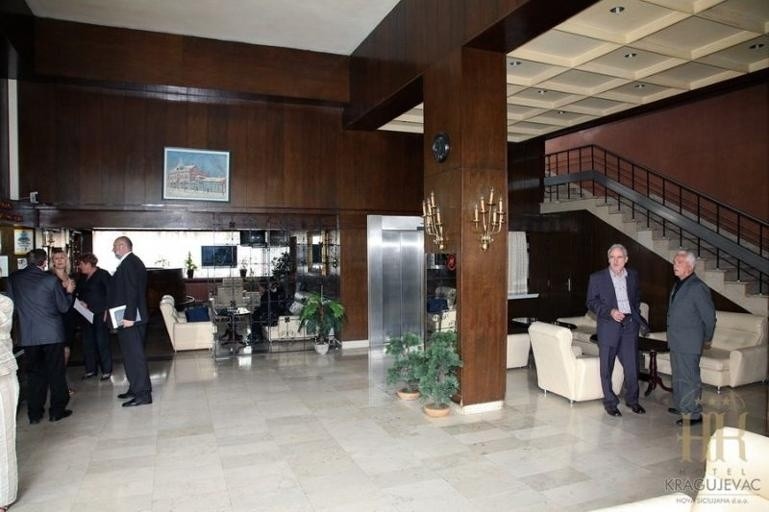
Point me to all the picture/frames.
[13,228,34,255]
[162,146,231,203]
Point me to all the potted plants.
[408,331,463,418]
[184,251,197,278]
[298,292,348,355]
[383,332,423,400]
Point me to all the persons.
[0,291,22,511]
[70,252,113,381]
[248,279,280,344]
[6,248,77,425]
[665,249,718,427]
[106,236,153,407]
[43,251,88,396]
[585,243,647,417]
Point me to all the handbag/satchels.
[184,301,211,323]
[426,295,449,314]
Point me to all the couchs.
[644,311,768,395]
[263,291,335,342]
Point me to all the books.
[107,304,141,329]
[72,297,95,325]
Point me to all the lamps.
[422,189,444,252]
[473,185,506,251]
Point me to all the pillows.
[185,306,210,322]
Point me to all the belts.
[623,313,633,318]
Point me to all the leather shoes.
[122,398,152,407]
[668,407,681,415]
[625,402,646,414]
[82,370,97,379]
[117,390,137,399]
[29,414,45,424]
[247,338,263,344]
[675,415,704,427]
[48,409,73,422]
[100,371,110,381]
[606,407,622,417]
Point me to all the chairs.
[559,302,650,355]
[585,425,769,512]
[160,295,217,352]
[528,321,625,405]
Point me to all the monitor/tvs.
[201,245,237,269]
